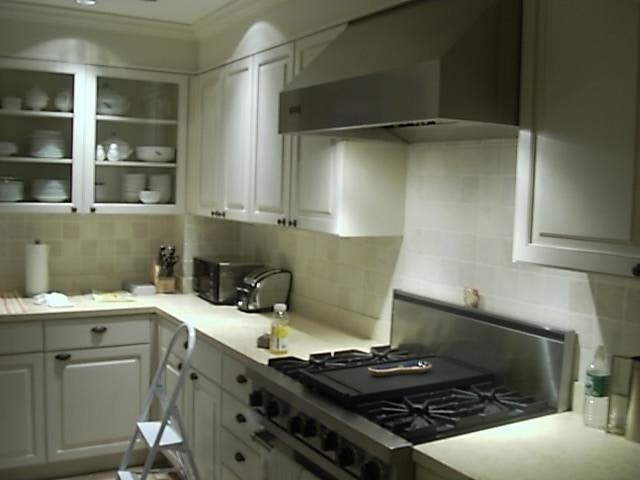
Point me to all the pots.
[96,84,130,117]
[28,128,69,159]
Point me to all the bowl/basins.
[32,177,72,204]
[1,173,27,201]
[136,146,175,162]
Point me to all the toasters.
[234,267,291,311]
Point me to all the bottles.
[106,133,130,159]
[97,144,119,162]
[607,399,624,435]
[578,349,610,429]
[268,303,291,354]
[25,82,72,112]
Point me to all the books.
[91,289,136,301]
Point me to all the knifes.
[158,243,179,270]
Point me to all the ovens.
[250,429,356,480]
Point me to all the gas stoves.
[249,345,548,478]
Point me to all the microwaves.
[192,255,263,305]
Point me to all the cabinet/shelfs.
[0,317,44,479]
[219,349,267,480]
[192,56,252,226]
[43,315,158,478]
[253,25,406,241]
[156,317,219,480]
[510,1,639,282]
[0,54,190,215]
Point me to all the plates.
[122,172,171,203]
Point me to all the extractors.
[277,0,519,147]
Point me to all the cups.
[4,96,20,110]
[624,355,639,443]
[2,141,18,158]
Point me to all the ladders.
[115,322,200,480]
